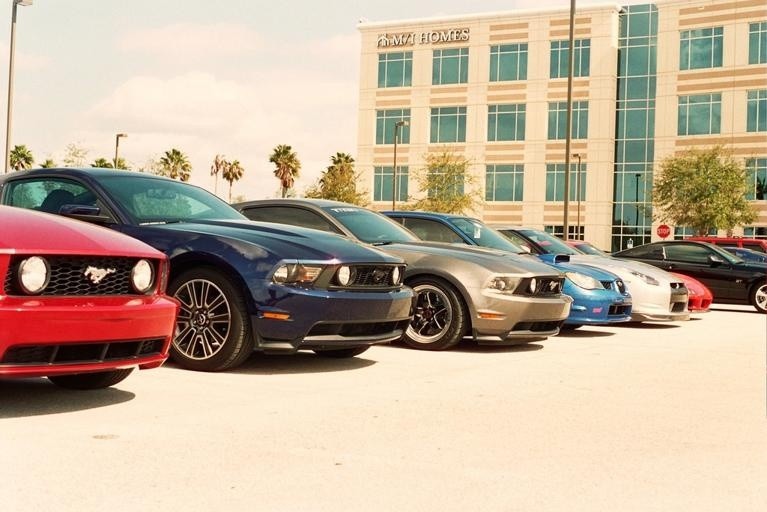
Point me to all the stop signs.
[657,225,670,238]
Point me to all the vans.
[682,236,766,255]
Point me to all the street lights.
[392,121,410,212]
[634,173,641,246]
[3,0,39,173]
[572,152,583,241]
[114,133,129,169]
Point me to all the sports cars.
[0,166,415,377]
[536,242,712,315]
[192,196,573,352]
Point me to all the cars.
[722,246,766,267]
[1,200,181,395]
[496,227,691,323]
[612,240,766,316]
[383,209,635,331]
